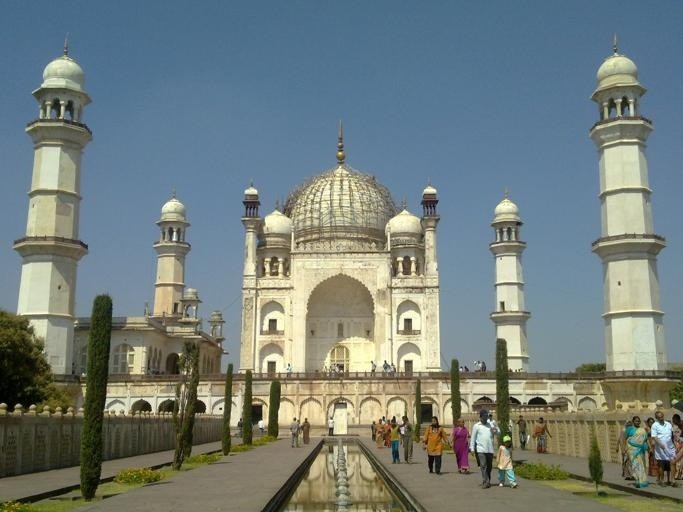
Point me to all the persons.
[289,417,310,447]
[371,360,396,377]
[328,417,334,435]
[258,418,264,435]
[459,360,486,373]
[371,416,415,464]
[286,364,292,377]
[616,411,682,487]
[239,418,243,438]
[508,368,523,372]
[335,364,340,374]
[422,415,450,474]
[451,410,553,488]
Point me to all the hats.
[479,410,489,417]
[503,436,512,443]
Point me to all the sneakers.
[457,467,470,474]
[481,480,519,490]
